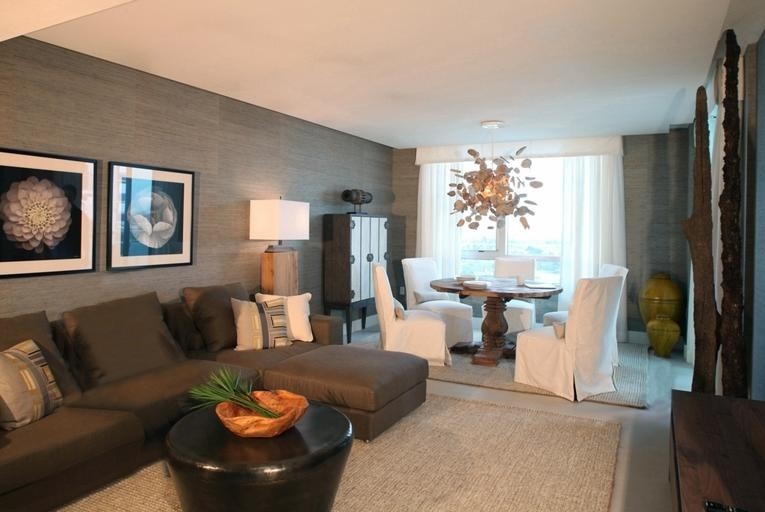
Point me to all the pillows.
[0,337,64,432]
[61,291,188,386]
[254,291,315,342]
[0,310,82,404]
[183,281,251,354]
[229,296,294,352]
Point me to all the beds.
[162,301,429,444]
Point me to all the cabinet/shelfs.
[322,214,406,344]
[668,390,765,512]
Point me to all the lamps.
[249,196,310,296]
[445,119,544,231]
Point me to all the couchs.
[0,297,263,512]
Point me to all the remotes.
[703,499,751,512]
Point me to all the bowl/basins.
[216,387,310,438]
[462,281,493,290]
[456,275,476,284]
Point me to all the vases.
[647,315,679,358]
[639,274,685,326]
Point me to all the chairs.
[372,255,629,394]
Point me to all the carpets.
[54,391,622,512]
[342,323,652,409]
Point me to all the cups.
[516,274,525,286]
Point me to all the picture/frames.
[0,146,98,279]
[105,161,194,272]
